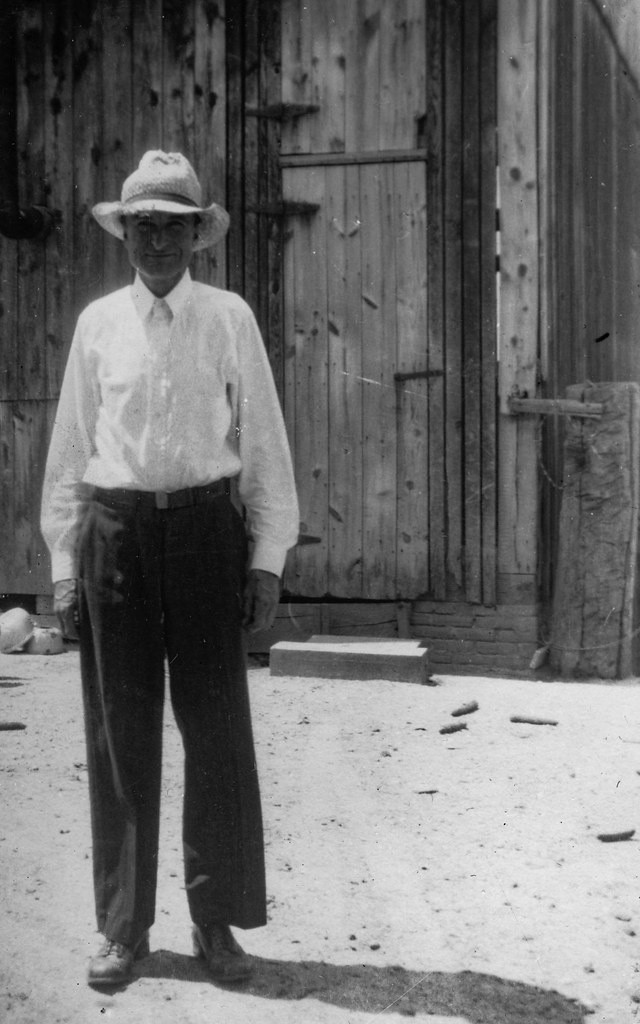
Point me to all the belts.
[94,479,226,509]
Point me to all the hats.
[92,149,229,253]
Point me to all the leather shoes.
[191,923,253,981]
[88,929,150,983]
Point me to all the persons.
[40,151,300,984]
